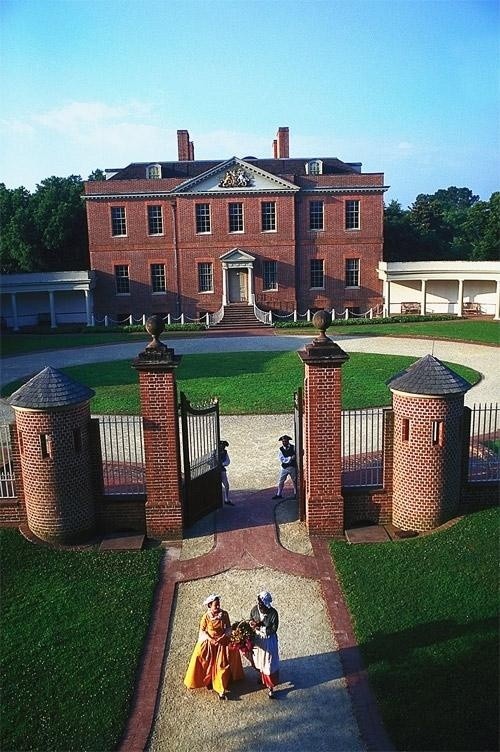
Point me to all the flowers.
[228,623,257,658]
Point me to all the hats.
[258,590,272,608]
[218,440,229,447]
[203,594,222,608]
[278,435,293,441]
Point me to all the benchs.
[462,302,482,315]
[401,302,422,314]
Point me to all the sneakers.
[224,500,235,506]
[272,495,282,499]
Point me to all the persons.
[249,590,280,699]
[271,435,296,500]
[207,440,235,506]
[183,591,245,699]
